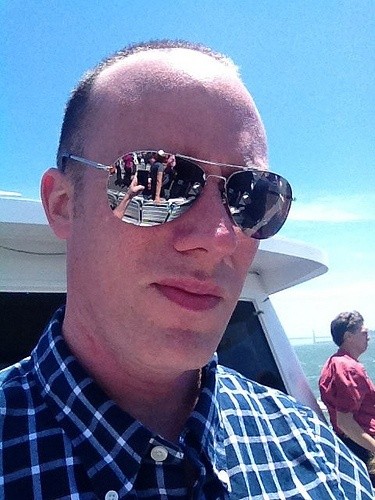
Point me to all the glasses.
[54,149,297,240]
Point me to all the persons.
[109,150,180,224]
[318,311,374,486]
[228,172,278,240]
[0,41,374,500]
